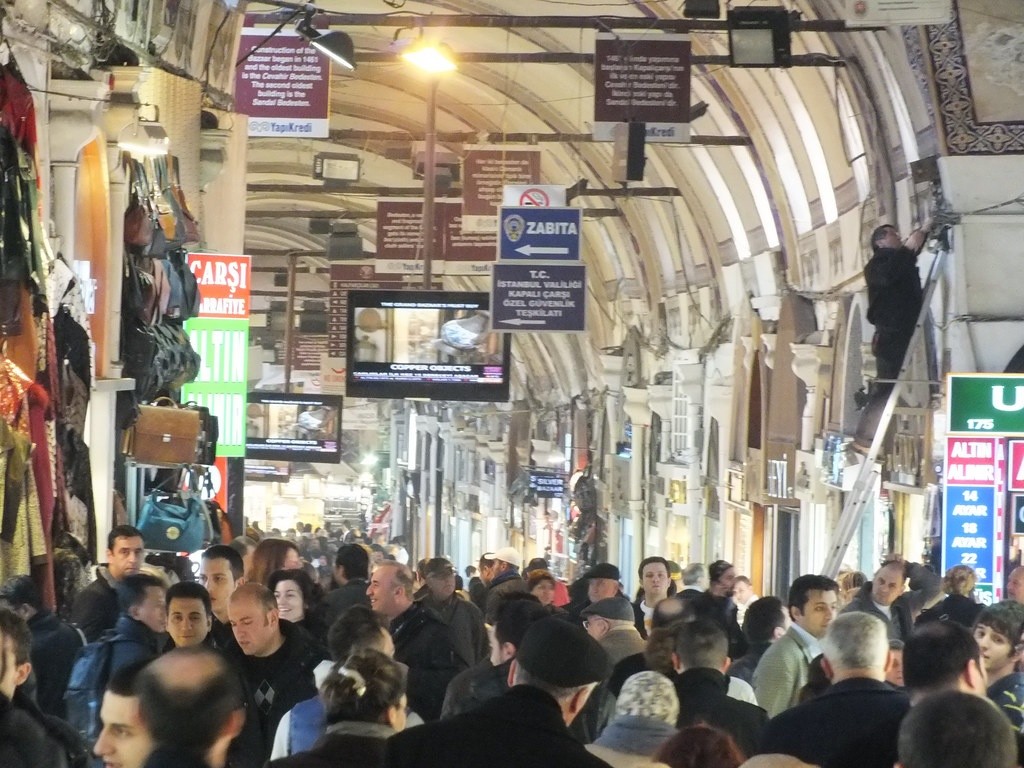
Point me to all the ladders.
[820,248,948,579]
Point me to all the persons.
[847,216,932,465]
[0,517,1024,768]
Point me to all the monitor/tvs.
[346,289,511,403]
[245,392,343,465]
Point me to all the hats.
[708,560,733,579]
[516,615,608,688]
[525,559,548,569]
[584,563,619,580]
[581,597,635,621]
[422,558,454,578]
[484,546,519,566]
[616,672,677,729]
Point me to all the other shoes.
[848,440,887,461]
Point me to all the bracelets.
[919,228,928,237]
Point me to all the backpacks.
[64,634,155,745]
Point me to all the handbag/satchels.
[131,398,201,466]
[136,474,203,554]
[182,401,218,468]
[121,154,202,392]
[202,488,232,546]
